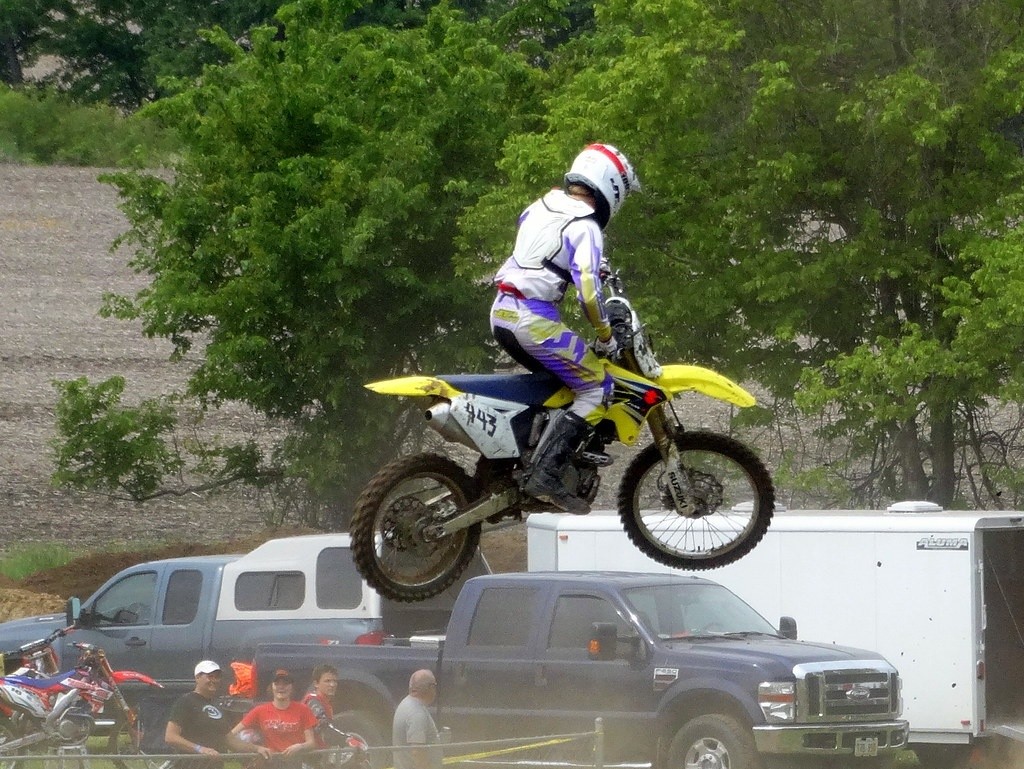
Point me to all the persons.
[487,143,645,517]
[301,663,370,769]
[391,667,445,769]
[163,658,273,769]
[225,669,318,769]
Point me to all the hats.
[194,660,221,676]
[271,669,292,684]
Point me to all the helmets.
[329,735,367,762]
[565,145,643,229]
[235,728,262,745]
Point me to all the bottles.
[441,726,451,743]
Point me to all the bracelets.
[194,744,203,753]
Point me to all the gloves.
[595,336,618,355]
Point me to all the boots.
[520,412,592,515]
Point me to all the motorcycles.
[1,610,176,769]
[352,263,775,601]
[2,624,89,769]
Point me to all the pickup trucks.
[254,571,909,769]
[1,531,492,752]
